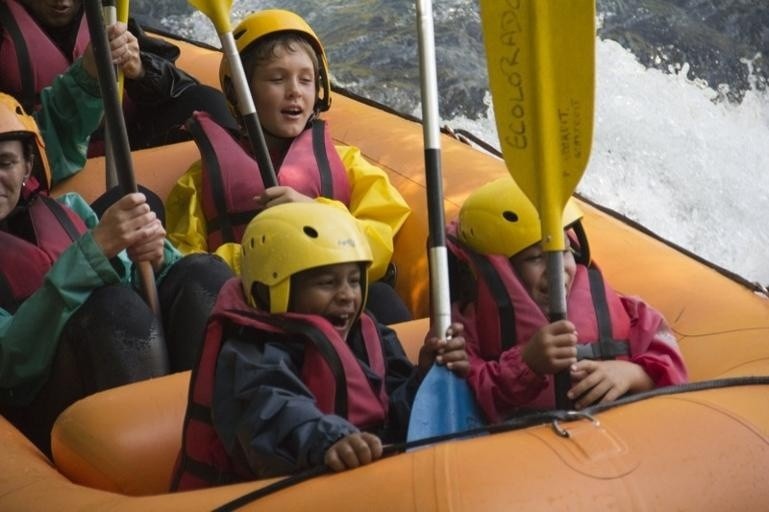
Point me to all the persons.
[440,176,689,426]
[0,85,237,463]
[170,200,472,495]
[0,0,244,161]
[167,5,415,334]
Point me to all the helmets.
[458,175,584,259]
[0,92,52,192]
[240,201,373,321]
[218,10,331,125]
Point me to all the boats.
[0,24,762,512]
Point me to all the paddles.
[406,0,492,451]
[480,0,596,411]
